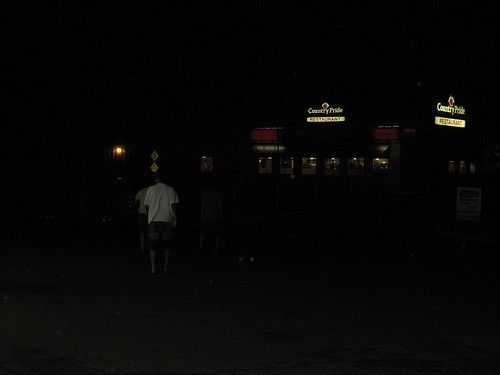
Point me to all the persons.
[133,180,154,253]
[144,171,180,275]
[196,178,224,254]
